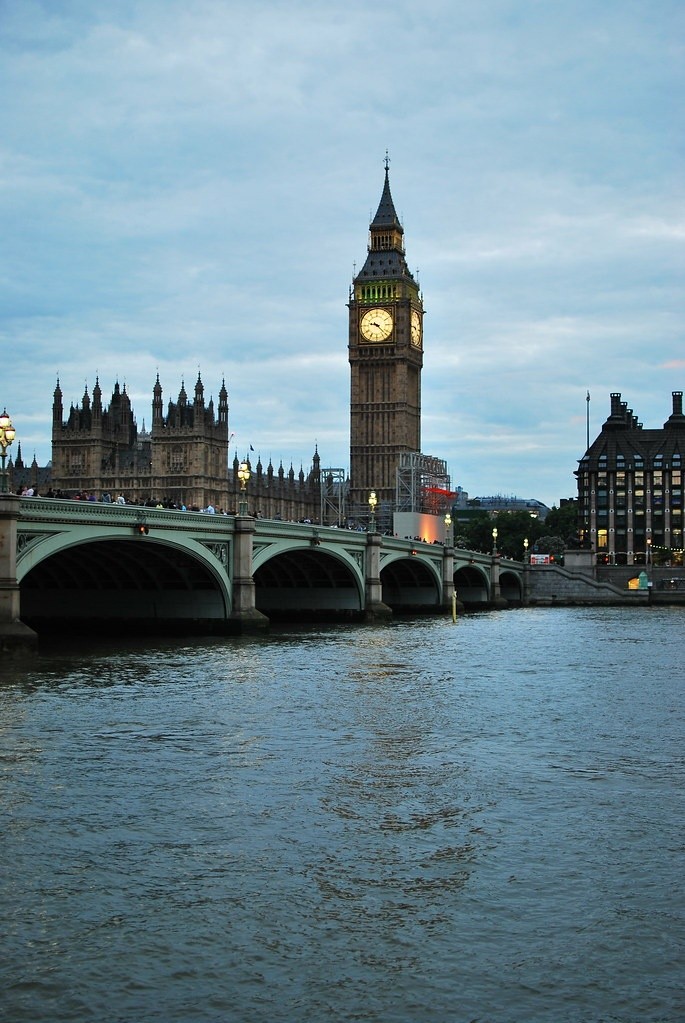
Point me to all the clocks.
[411,312,421,345]
[360,308,393,340]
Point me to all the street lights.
[646,536,652,564]
[523,537,529,562]
[0,406,15,492]
[444,513,452,546]
[237,458,251,515]
[368,491,377,532]
[492,526,499,555]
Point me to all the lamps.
[471,559,475,563]
[411,549,417,555]
[137,524,149,535]
[312,537,321,545]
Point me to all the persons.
[74,490,96,501]
[274,512,282,520]
[227,508,236,515]
[404,535,445,546]
[200,506,215,514]
[46,488,72,499]
[167,497,187,512]
[383,529,398,538]
[125,498,144,506]
[98,490,125,505]
[248,510,263,520]
[294,515,314,524]
[500,553,515,561]
[145,497,167,509]
[454,545,492,556]
[315,516,330,526]
[186,502,200,512]
[214,504,227,516]
[16,485,41,497]
[331,518,367,532]
